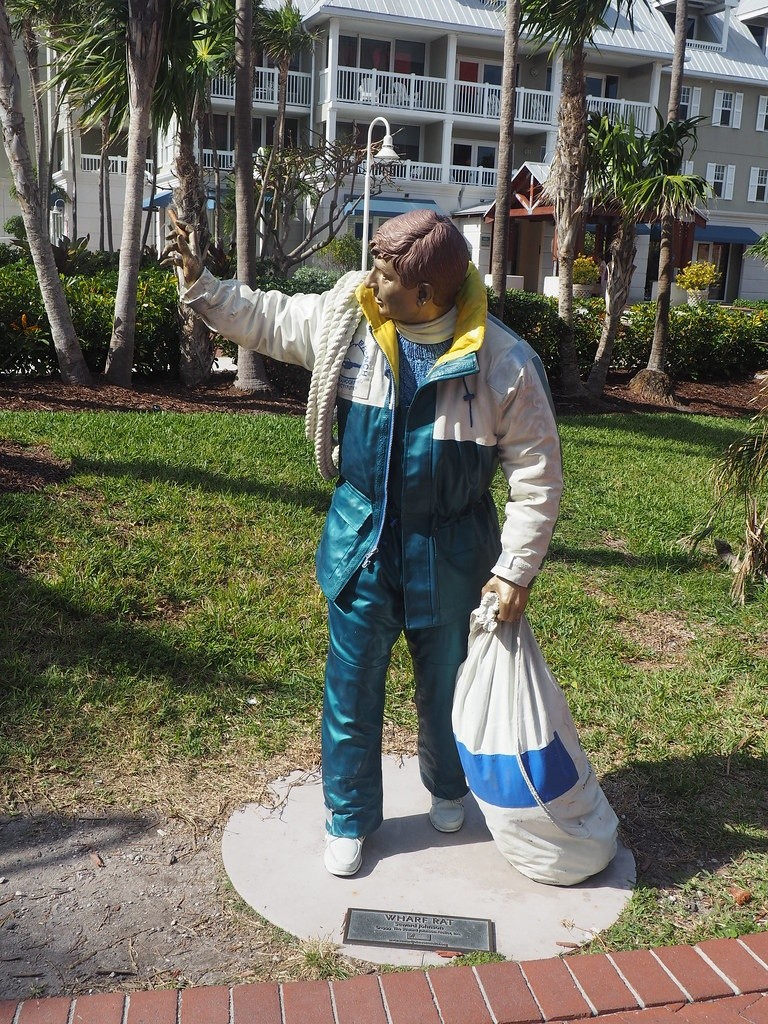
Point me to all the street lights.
[52,199,68,239]
[254,147,265,257]
[361,116,402,270]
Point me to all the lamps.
[51,198,66,215]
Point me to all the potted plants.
[573,253,602,298]
[676,261,722,305]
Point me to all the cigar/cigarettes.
[167,209,188,240]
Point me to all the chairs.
[488,94,499,114]
[357,77,381,106]
[393,82,419,106]
[532,99,546,120]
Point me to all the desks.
[382,94,395,97]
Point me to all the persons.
[161,209,564,877]
